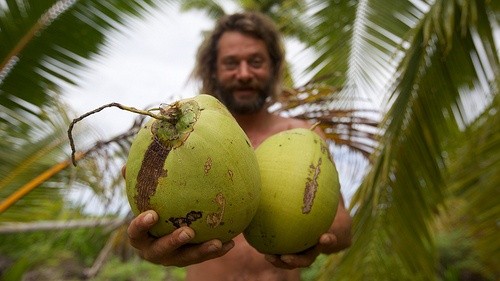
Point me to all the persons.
[122,12,352,281]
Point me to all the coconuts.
[125,95,260,245]
[241,128,340,255]
